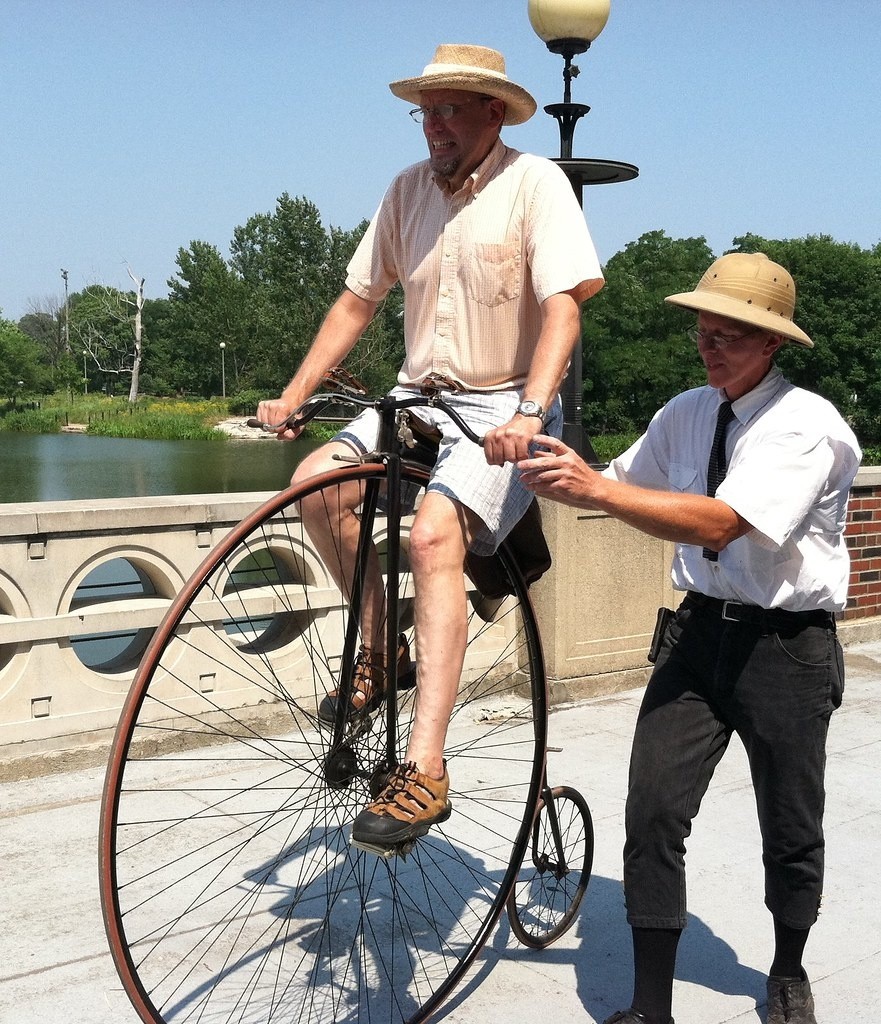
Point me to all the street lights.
[220,342,226,398]
[82,351,87,393]
[526,0,642,467]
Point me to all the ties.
[702,400,735,560]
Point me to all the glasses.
[684,321,756,344]
[411,101,487,122]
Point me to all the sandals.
[354,757,450,845]
[318,635,418,722]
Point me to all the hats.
[663,254,814,348]
[388,45,534,125]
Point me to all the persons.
[516,250,862,1024]
[257,43,606,845]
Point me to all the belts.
[680,588,833,631]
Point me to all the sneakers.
[767,963,816,1024]
[604,1008,674,1024]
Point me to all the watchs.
[515,400,547,423]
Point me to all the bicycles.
[97,365,596,1024]
